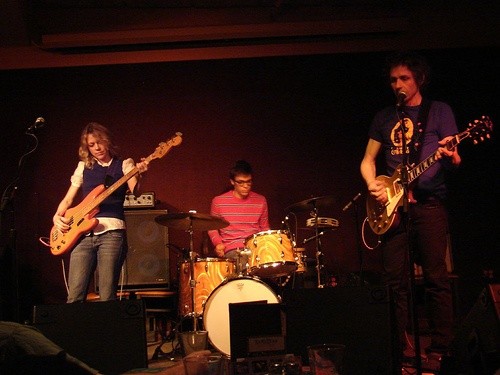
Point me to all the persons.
[360,54,461,369]
[53,122,148,303]
[208,159,269,265]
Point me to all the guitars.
[48,133,183,256]
[366,114,495,235]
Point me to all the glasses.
[232,179,252,184]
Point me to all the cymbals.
[153,211,231,232]
[277,194,338,214]
[306,217,339,229]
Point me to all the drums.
[242,230,298,279]
[202,275,283,355]
[290,245,312,275]
[174,256,239,320]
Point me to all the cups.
[307,343,347,375]
[181,330,208,356]
[182,354,221,375]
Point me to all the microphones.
[25,117,45,133]
[172,244,189,257]
[397,89,407,107]
[298,230,327,246]
[280,214,289,230]
[342,193,361,212]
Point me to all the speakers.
[94,210,171,293]
[355,200,385,275]
[33,296,148,375]
[282,284,402,375]
[450,283,500,375]
